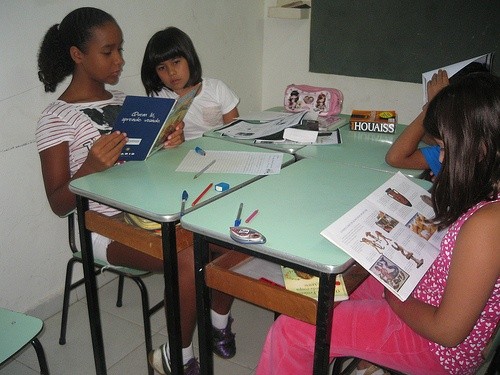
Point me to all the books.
[349,109,397,134]
[256,119,320,143]
[111,83,201,161]
[321,172,458,304]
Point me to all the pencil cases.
[284,84,344,117]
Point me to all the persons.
[33,5,238,374]
[254,61,500,374]
[384,60,488,176]
[137,26,241,151]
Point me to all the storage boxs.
[350,110,396,134]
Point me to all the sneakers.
[211,310,236,359]
[148,342,200,375]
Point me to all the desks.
[295,124,427,179]
[203,107,352,154]
[180,158,434,375]
[68,136,295,375]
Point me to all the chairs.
[0,307,49,375]
[332,324,500,375]
[59,210,165,375]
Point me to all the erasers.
[215,182,229,192]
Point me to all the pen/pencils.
[234,202,243,227]
[193,159,216,179]
[191,183,214,206]
[180,190,188,216]
[245,209,260,223]
